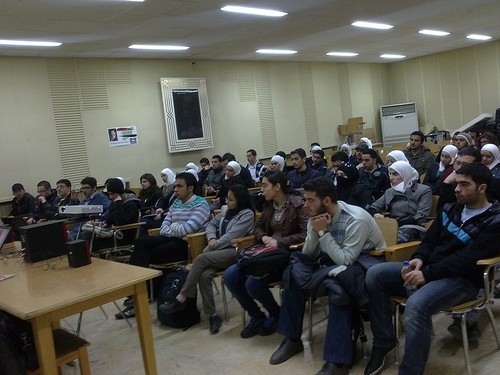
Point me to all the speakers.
[64,240,91,268]
[18,219,68,263]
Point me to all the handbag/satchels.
[81,220,124,239]
[236,242,289,280]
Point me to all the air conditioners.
[380,102,420,148]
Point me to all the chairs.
[64,116,500,375]
[0,310,92,375]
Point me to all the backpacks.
[156,267,201,331]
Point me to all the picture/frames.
[160,77,213,154]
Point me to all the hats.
[107,179,124,194]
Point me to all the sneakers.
[159,297,187,313]
[364,333,400,375]
[209,315,222,332]
[314,361,351,375]
[270,337,304,364]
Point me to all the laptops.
[0,226,12,250]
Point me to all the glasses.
[13,189,24,195]
[37,187,50,194]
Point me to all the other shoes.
[260,307,281,336]
[115,305,136,319]
[447,317,482,339]
[240,312,266,337]
[123,298,134,305]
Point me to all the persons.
[169,183,256,334]
[223,169,311,338]
[269,175,387,375]
[114,171,210,320]
[7,130,500,375]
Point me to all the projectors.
[58,205,104,214]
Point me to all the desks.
[0,240,162,375]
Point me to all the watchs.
[317,229,329,239]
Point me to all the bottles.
[403,261,417,297]
